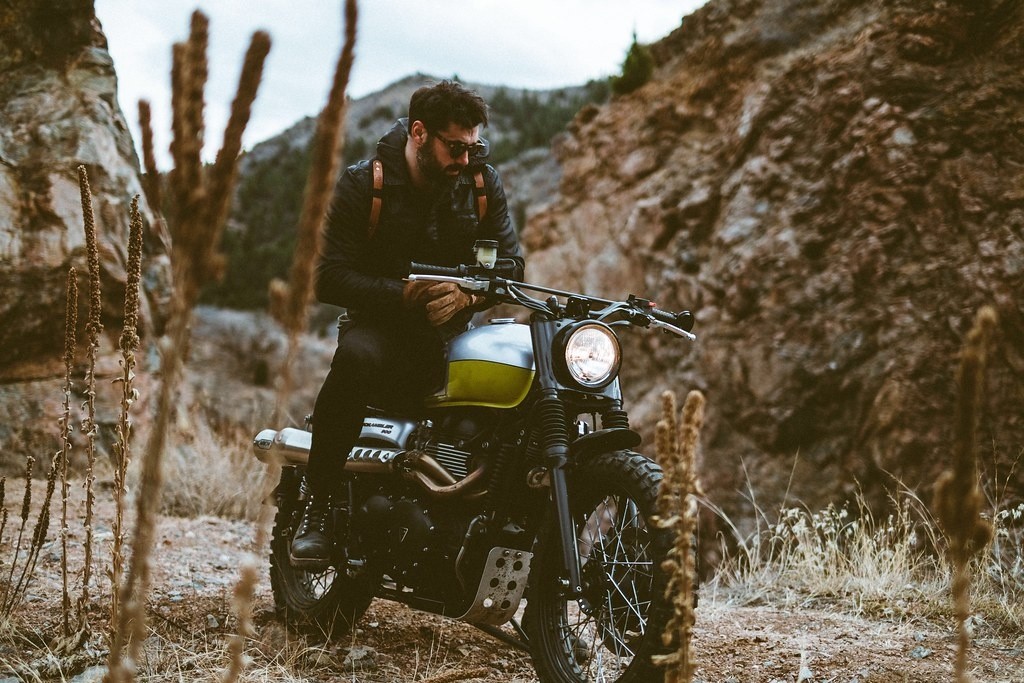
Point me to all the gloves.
[425,280,487,327]
[403,280,440,309]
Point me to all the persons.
[288,79,589,665]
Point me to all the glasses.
[418,119,486,159]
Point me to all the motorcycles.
[253,236,700,683]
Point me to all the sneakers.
[521,598,590,664]
[290,494,335,570]
